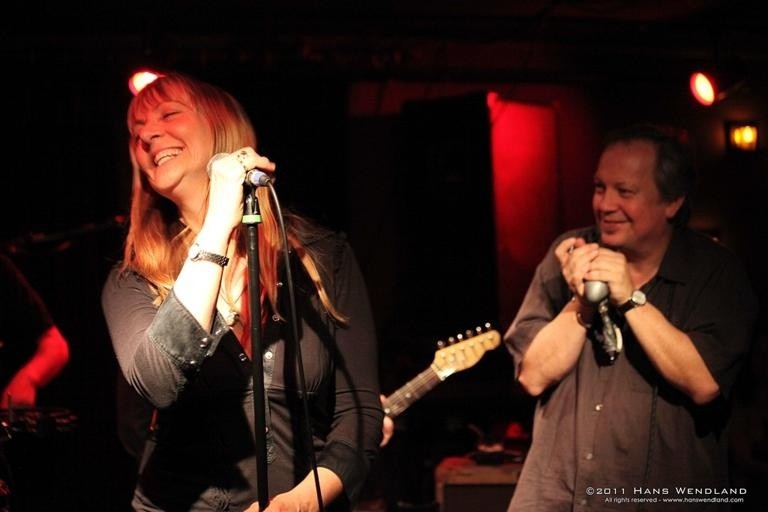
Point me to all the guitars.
[386,323,501,416]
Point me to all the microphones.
[206,152,271,190]
[583,280,617,364]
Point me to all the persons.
[503,130,757,512]
[378,395,395,448]
[0,248,74,417]
[100,70,383,512]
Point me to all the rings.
[236,149,248,161]
[239,160,248,171]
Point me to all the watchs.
[188,242,229,267]
[618,289,647,315]
